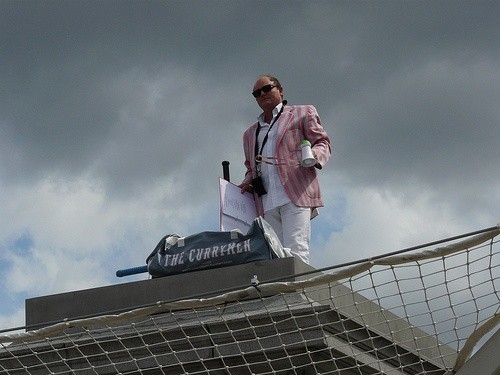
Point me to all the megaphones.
[300,140,317,168]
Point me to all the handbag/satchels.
[146,216,285,279]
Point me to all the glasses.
[251,84,277,97]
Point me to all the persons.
[238,74,332,263]
